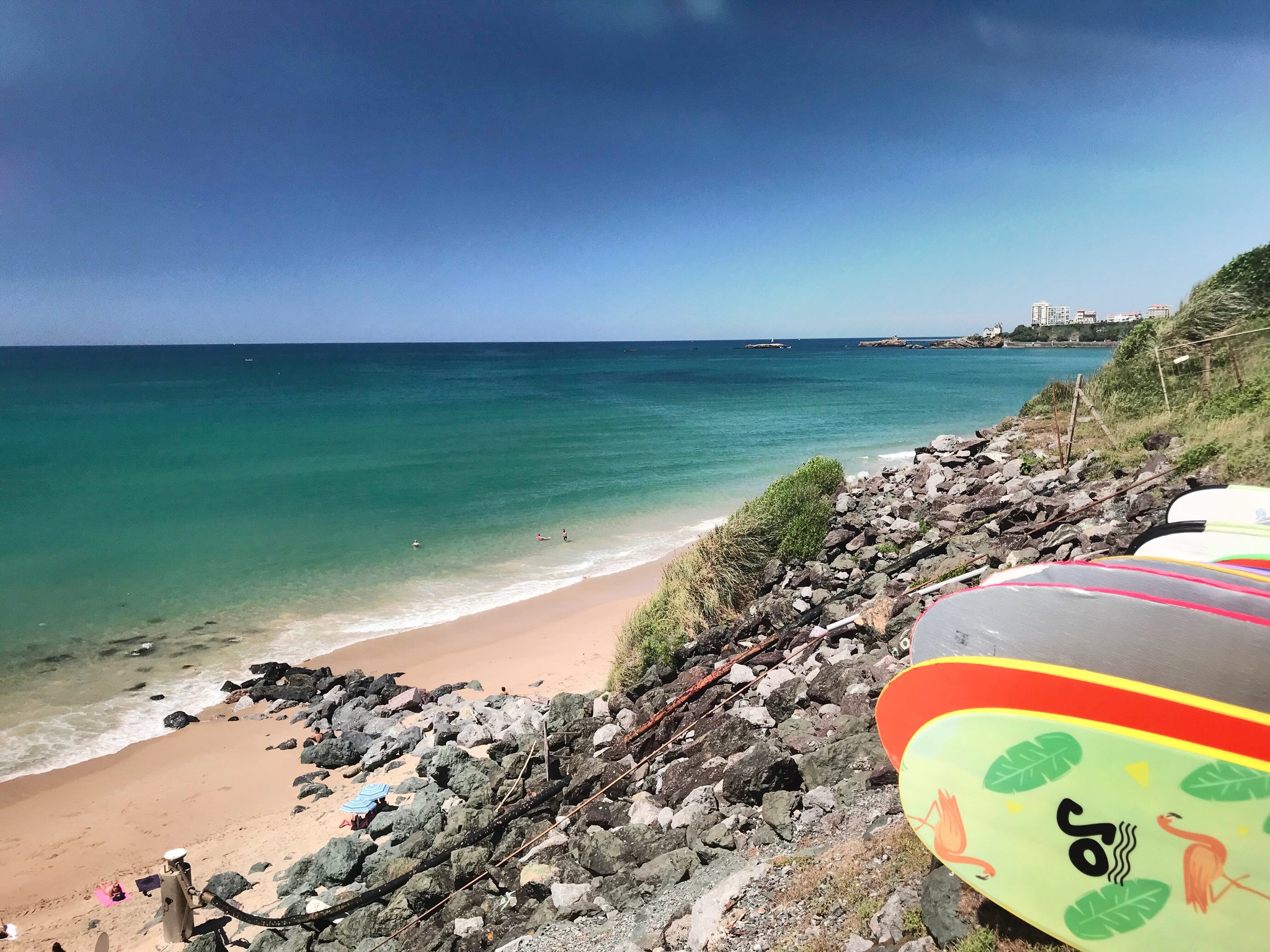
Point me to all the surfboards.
[875,483,1270,951]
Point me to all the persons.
[412,540,420,546]
[0,917,12,939]
[499,687,508,695]
[538,534,551,540]
[96,878,122,899]
[375,796,388,812]
[310,727,323,745]
[52,942,66,952]
[562,529,567,541]
[337,812,370,828]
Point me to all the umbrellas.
[339,798,377,829]
[357,783,392,815]
